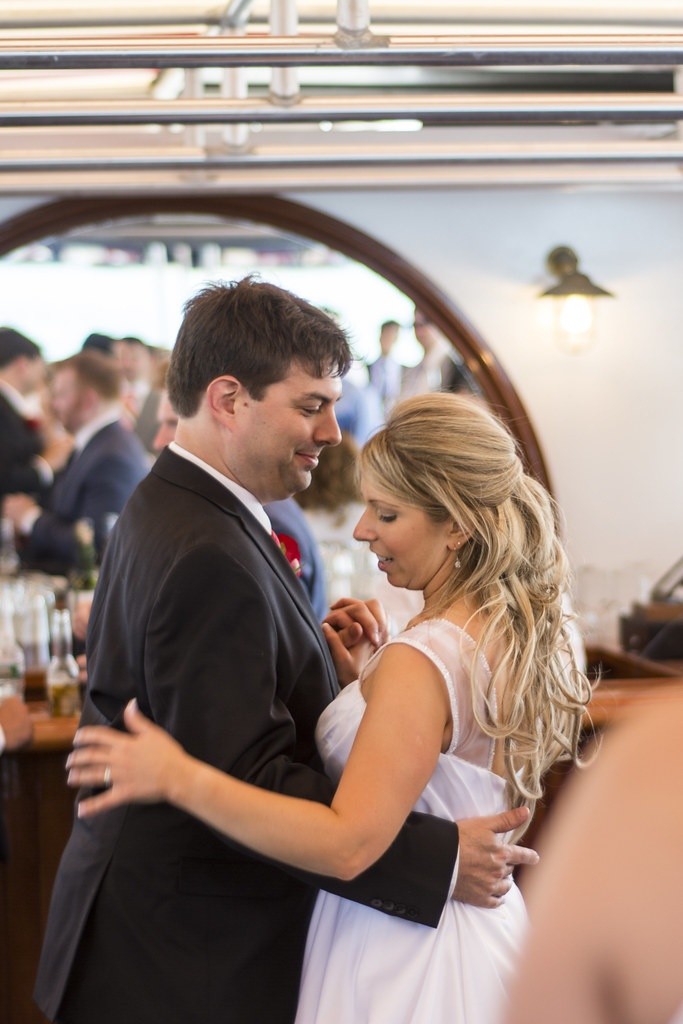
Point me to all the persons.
[65,389,609,1024]
[0,301,491,625]
[29,276,542,1024]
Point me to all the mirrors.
[0,190,562,697]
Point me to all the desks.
[0,677,672,1024]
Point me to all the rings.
[103,765,114,784]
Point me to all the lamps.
[150,0,372,154]
[540,244,614,337]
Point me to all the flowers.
[271,531,302,577]
[23,414,48,436]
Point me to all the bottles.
[0,606,26,705]
[0,518,21,577]
[65,517,99,660]
[44,607,81,718]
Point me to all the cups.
[0,678,23,705]
